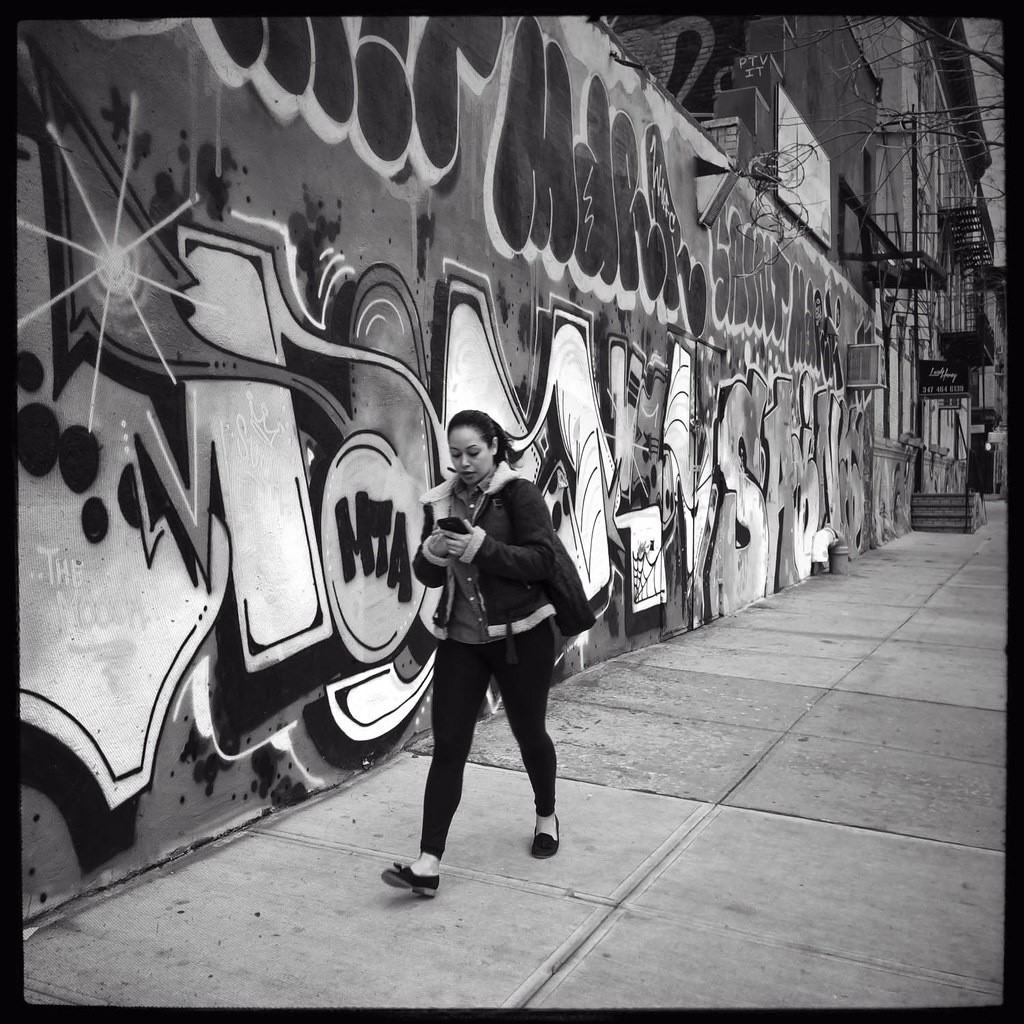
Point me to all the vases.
[930,444,939,453]
[909,437,924,450]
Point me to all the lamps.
[827,316,840,337]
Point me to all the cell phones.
[436,516,469,541]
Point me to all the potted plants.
[900,431,914,444]
[939,447,950,456]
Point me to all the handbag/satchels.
[504,478,597,636]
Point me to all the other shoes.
[531,813,559,859]
[380,867,440,898]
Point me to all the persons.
[381,408,562,900]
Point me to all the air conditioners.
[846,343,887,390]
